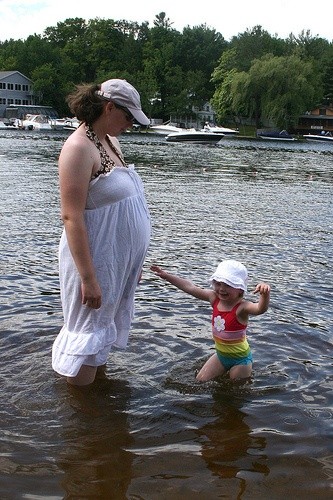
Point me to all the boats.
[302,133,333,143]
[147,124,195,136]
[256,131,296,141]
[198,126,239,136]
[24,113,79,131]
[164,131,224,146]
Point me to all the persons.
[49,77,152,388]
[149,259,270,384]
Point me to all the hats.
[209,259,248,295]
[97,79,150,126]
